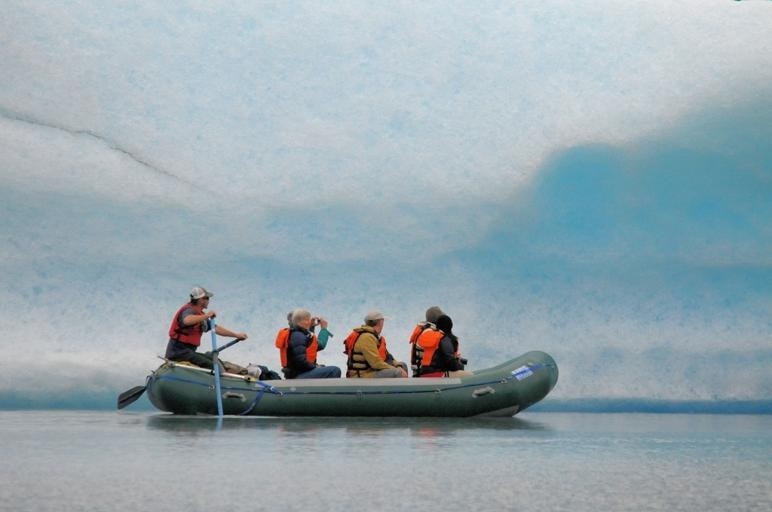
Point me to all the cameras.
[456,356,468,365]
[317,320,322,323]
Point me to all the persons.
[283,309,342,378]
[409,306,464,376]
[275,311,333,372]
[417,314,472,378]
[165,286,248,374]
[342,310,407,378]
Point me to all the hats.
[190,287,213,299]
[425,306,447,322]
[367,308,384,320]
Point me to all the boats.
[147,349,558,417]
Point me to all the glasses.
[202,297,210,300]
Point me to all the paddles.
[118,339,245,409]
